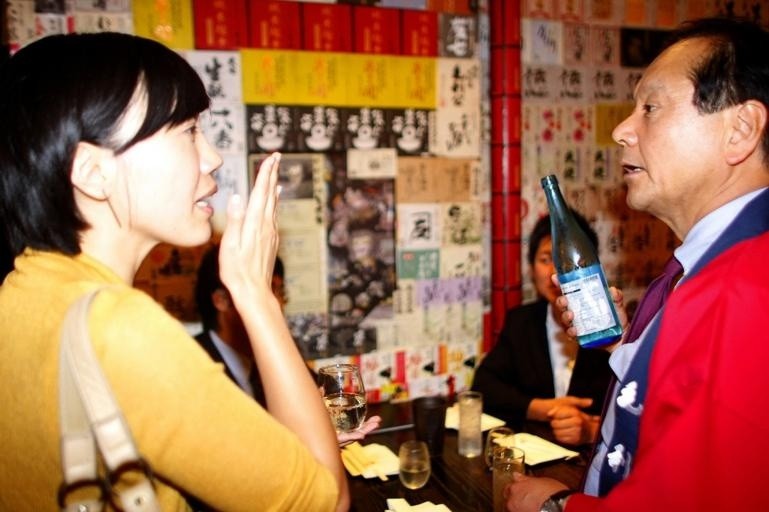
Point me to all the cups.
[492,449,526,511]
[485,429,515,470]
[457,392,482,460]
[399,442,431,504]
[319,363,367,432]
[411,397,446,456]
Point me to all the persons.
[191,244,337,415]
[553,14,767,511]
[472,207,616,445]
[3,31,381,512]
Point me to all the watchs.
[540,487,579,512]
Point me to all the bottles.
[541,174,622,345]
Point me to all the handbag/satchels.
[59,286,163,512]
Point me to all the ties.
[576,250,683,498]
